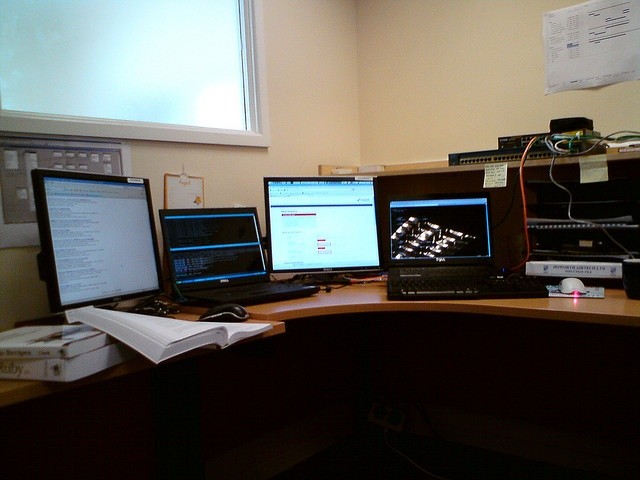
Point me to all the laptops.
[385,191,496,264]
[156,205,321,306]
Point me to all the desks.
[163,277,640,326]
[1,304,287,408]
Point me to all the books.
[0,325,113,359]
[0,344,140,383]
[74,307,272,364]
[525,260,622,279]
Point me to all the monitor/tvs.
[30,167,166,316]
[262,175,388,285]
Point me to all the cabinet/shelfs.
[319,151,640,278]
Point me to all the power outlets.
[372,404,407,434]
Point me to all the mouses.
[558,276,589,296]
[192,301,262,324]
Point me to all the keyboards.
[385,268,553,304]
